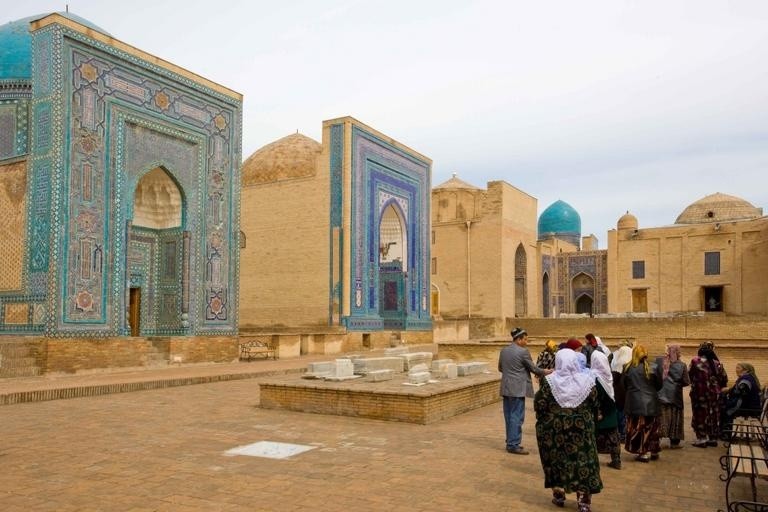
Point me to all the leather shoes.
[509,449,530,456]
[506,445,523,450]
[719,434,730,441]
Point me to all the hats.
[511,327,527,339]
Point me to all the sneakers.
[651,454,659,460]
[671,443,684,449]
[552,498,564,506]
[636,456,649,463]
[608,462,622,470]
[692,442,707,449]
[707,441,718,446]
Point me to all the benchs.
[715,431,768,512]
[720,386,768,472]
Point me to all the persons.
[537,333,690,470]
[534,348,604,511]
[499,327,552,454]
[709,296,716,311]
[688,342,762,449]
